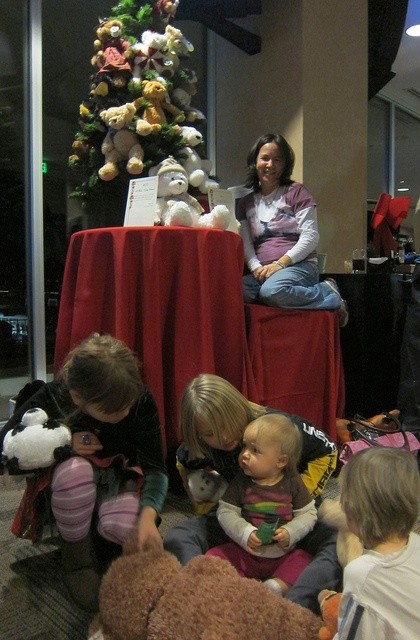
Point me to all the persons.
[1,332,169,614]
[242,133,350,329]
[204,413,319,597]
[162,373,342,614]
[330,446,420,640]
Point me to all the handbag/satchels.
[336,409,409,472]
[338,431,420,465]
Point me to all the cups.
[352,247,366,274]
[318,253,327,273]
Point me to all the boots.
[60,534,102,613]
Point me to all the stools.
[246,306,346,446]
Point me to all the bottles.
[397,240,405,264]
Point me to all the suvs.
[2,287,29,365]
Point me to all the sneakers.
[324,277,349,327]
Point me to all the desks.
[52,227,244,459]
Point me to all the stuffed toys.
[98,102,153,181]
[163,25,189,71]
[173,125,220,194]
[127,30,174,92]
[316,477,363,568]
[169,67,207,124]
[148,155,231,230]
[188,467,229,504]
[314,588,344,640]
[85,526,321,640]
[90,19,135,88]
[132,80,186,136]
[2,407,72,471]
